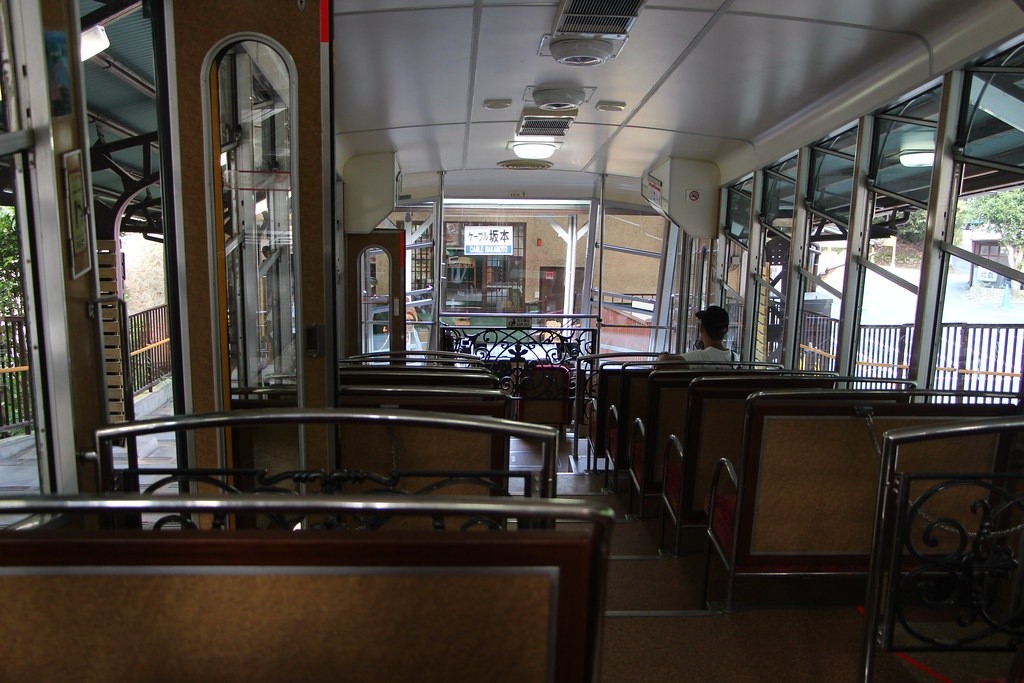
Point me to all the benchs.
[0,350,1024,683]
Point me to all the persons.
[654,305,741,370]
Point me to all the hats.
[695,305,729,328]
[262,246,270,252]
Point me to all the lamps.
[549,40,614,67]
[533,89,585,111]
[506,141,564,159]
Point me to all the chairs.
[515,365,571,439]
